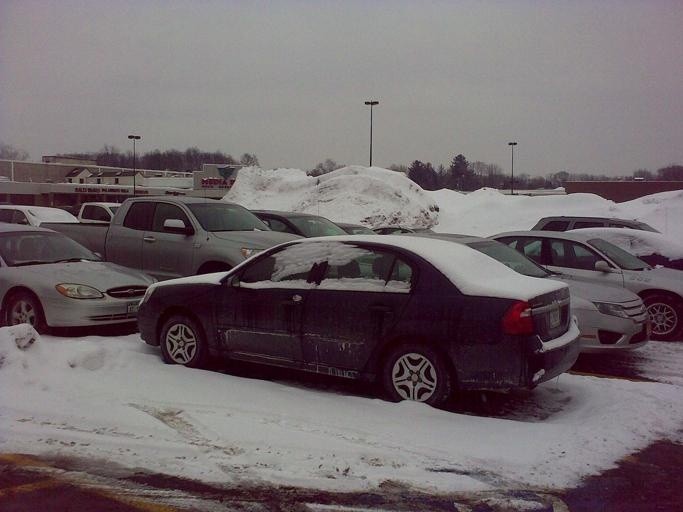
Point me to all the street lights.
[365,98,379,169]
[507,141,518,194]
[127,134,141,199]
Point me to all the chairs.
[338,258,399,280]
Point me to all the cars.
[0,204,84,226]
[0,221,158,334]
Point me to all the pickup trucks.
[41,198,307,280]
